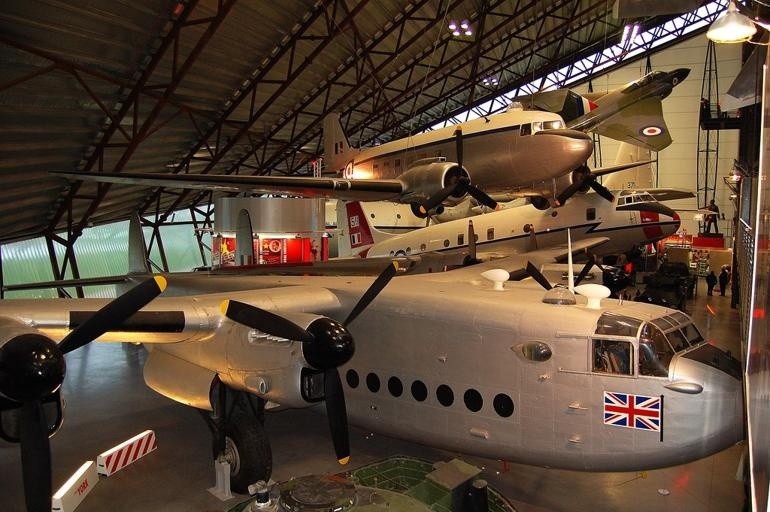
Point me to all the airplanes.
[328,184,698,265]
[48,66,691,227]
[0,221,769,511]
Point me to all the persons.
[705,270,718,296]
[704,199,721,234]
[718,266,728,297]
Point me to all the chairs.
[639,339,665,371]
[597,344,629,374]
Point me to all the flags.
[214,236,329,269]
[604,390,661,432]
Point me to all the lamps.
[448,19,472,37]
[706,0,770,46]
[482,77,499,87]
[728,158,750,181]
